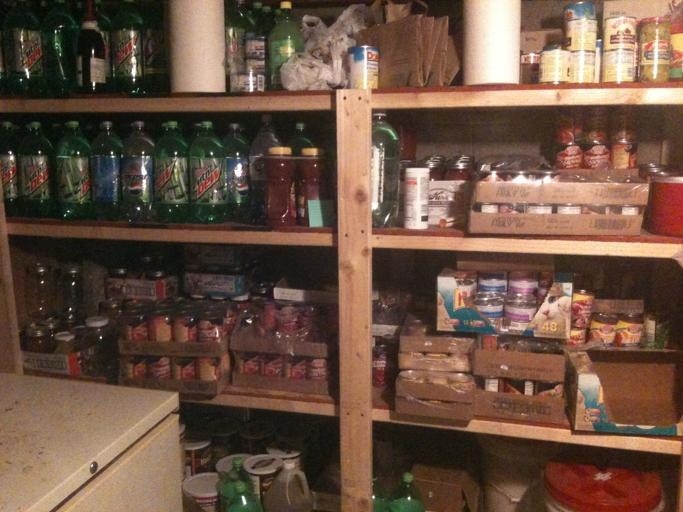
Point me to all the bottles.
[0,1,400,228]
[216,457,263,511]
[22,260,115,377]
[371,470,426,512]
[405,168,430,229]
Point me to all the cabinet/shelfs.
[0,80,683,512]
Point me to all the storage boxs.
[436,171,681,440]
[407,441,483,512]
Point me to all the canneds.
[229,34,266,92]
[521,1,683,84]
[423,105,683,228]
[22,244,338,383]
[347,44,378,89]
[372,273,670,405]
[179,414,309,512]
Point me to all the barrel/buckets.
[480,447,542,512]
[541,460,664,511]
[262,460,316,511]
